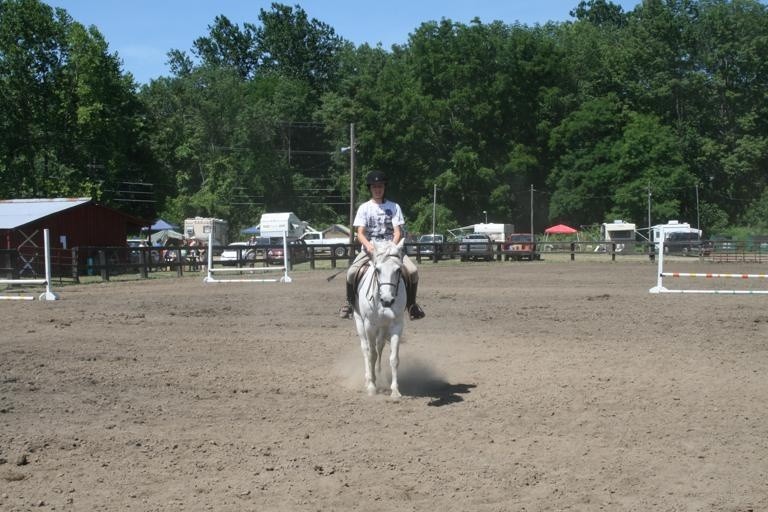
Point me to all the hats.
[365,171,388,187]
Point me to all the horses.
[350,237,410,400]
[163,234,204,255]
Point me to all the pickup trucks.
[503,233,540,261]
[459,234,495,261]
[298,232,358,258]
[416,234,458,259]
[666,232,712,257]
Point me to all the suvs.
[126,238,158,267]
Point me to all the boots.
[339,276,355,319]
[406,279,426,321]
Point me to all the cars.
[220,242,257,265]
[404,232,420,255]
[267,237,307,264]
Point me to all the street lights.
[482,211,488,224]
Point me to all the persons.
[339,170,424,321]
[135,237,206,272]
